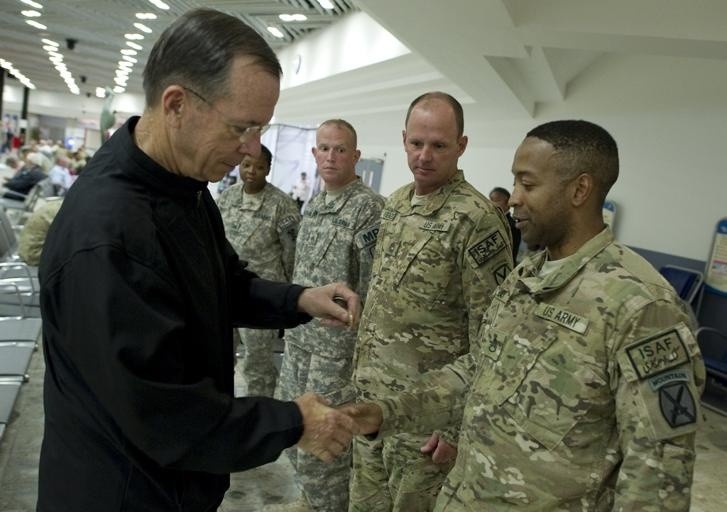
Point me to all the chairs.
[660,264,704,308]
[0,203,50,447]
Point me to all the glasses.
[184,86,270,143]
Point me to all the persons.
[489,189,520,264]
[275,121,389,512]
[36,7,362,511]
[1,113,91,265]
[219,140,299,397]
[334,120,706,512]
[350,90,515,512]
[290,172,310,208]
